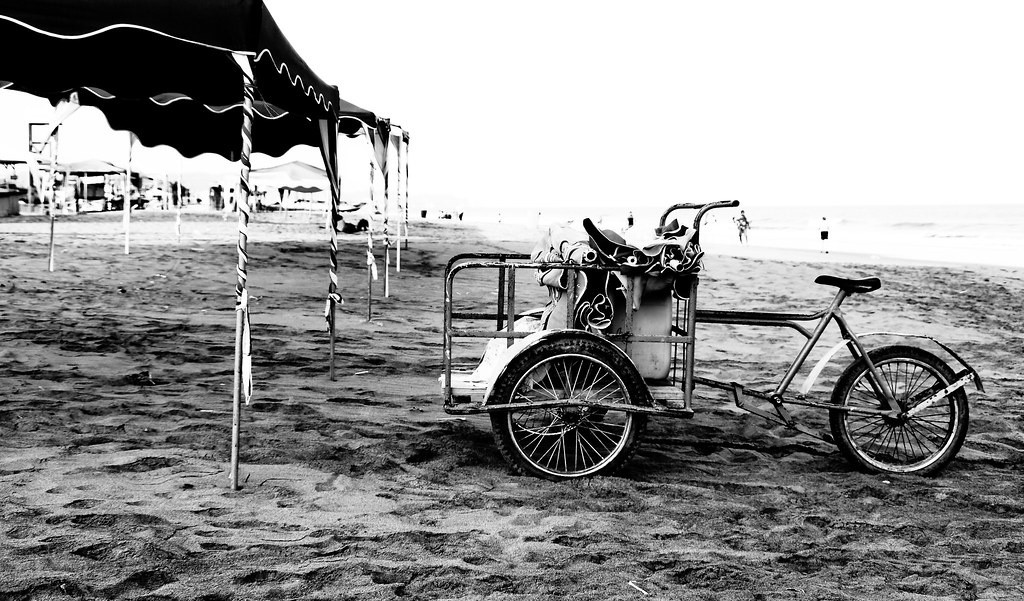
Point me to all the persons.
[734,209,752,243]
[817,216,831,253]
[627,210,634,227]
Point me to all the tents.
[0,1,410,492]
[243,159,342,230]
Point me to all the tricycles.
[438,199,984,479]
